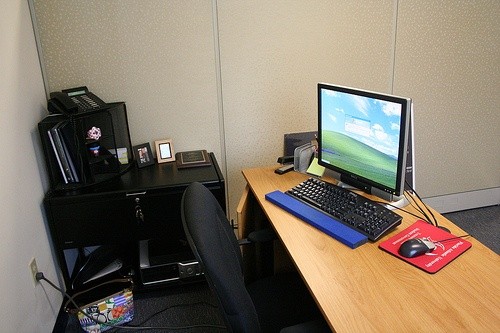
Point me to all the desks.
[43,152,226,297]
[235,165,500,333]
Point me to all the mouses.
[399,238,435,258]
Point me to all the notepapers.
[306,157,325,177]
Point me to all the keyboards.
[285,177,404,243]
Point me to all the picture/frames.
[176,150,212,169]
[154,138,176,164]
[132,142,156,168]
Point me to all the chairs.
[180,181,332,333]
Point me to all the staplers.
[274,156,294,175]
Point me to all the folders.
[47,120,80,184]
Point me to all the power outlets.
[28,257,41,286]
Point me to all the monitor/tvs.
[318,82,411,202]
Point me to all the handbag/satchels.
[77,287,134,332]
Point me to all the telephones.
[47,86,107,119]
